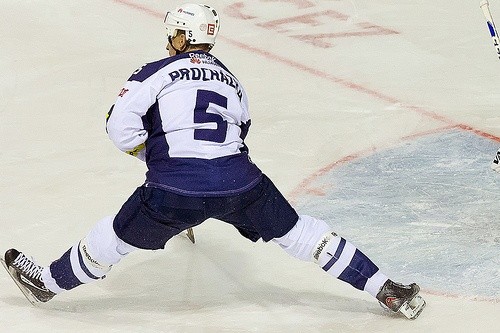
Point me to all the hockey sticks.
[480,0,500,59]
[175,226,197,245]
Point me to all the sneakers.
[376,279,426,321]
[490,148,500,174]
[1,248,57,307]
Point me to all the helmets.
[163,4,220,49]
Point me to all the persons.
[1,2,427,321]
